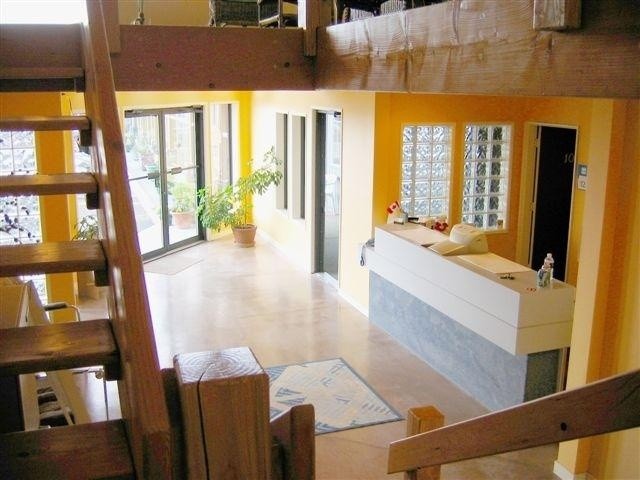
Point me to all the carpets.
[260,358,405,437]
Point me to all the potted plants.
[171,183,195,230]
[197,157,282,248]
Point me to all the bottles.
[544,253,555,281]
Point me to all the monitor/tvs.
[427,223,488,255]
